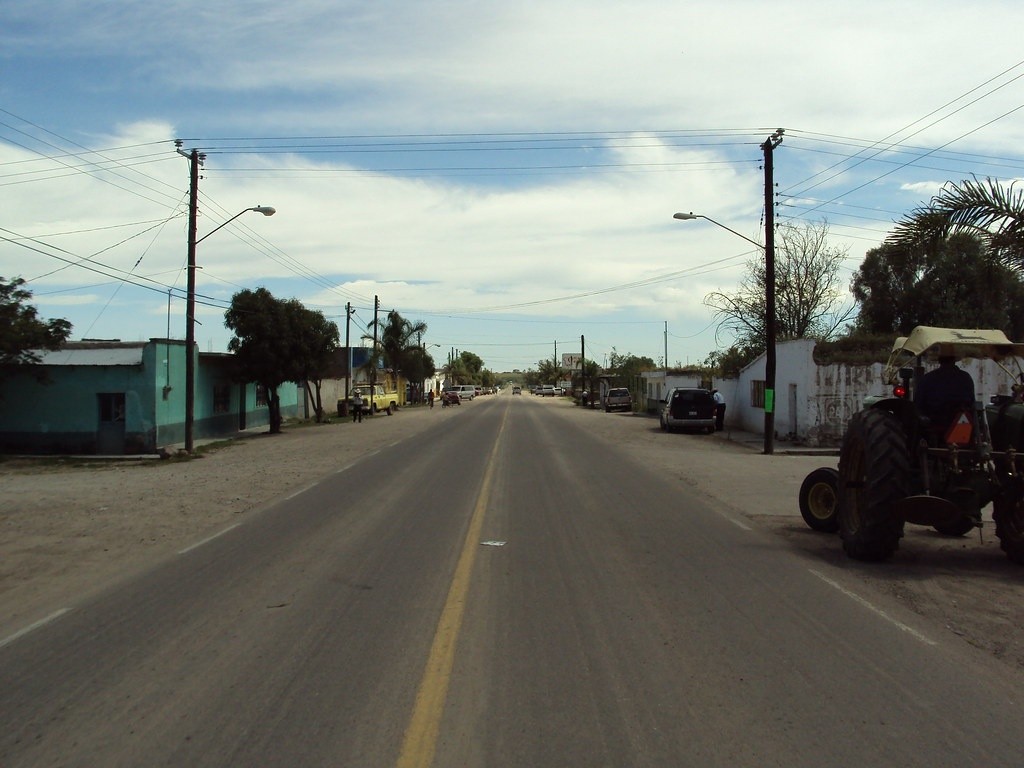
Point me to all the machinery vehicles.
[798,326,1024,573]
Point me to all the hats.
[354,389,362,393]
[934,344,959,357]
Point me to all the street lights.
[174,138,275,456]
[423,343,441,402]
[673,129,783,454]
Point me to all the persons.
[917,353,977,411]
[428,389,434,408]
[712,390,726,432]
[581,390,588,406]
[444,393,450,406]
[352,390,364,423]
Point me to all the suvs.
[660,386,718,435]
[603,388,633,413]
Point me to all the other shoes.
[353,419,356,422]
[359,419,362,423]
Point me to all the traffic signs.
[562,353,582,369]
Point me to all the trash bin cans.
[337,399,348,417]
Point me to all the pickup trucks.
[337,379,399,416]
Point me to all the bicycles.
[442,398,453,408]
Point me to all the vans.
[446,385,475,400]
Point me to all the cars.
[475,381,513,395]
[447,392,462,405]
[512,384,566,398]
